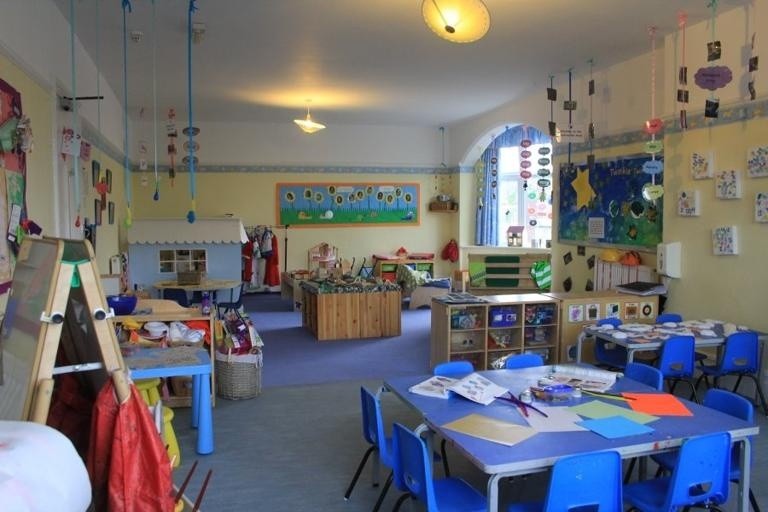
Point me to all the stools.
[147,403,182,466]
[407,277,452,310]
[132,377,163,405]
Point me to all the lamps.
[420,1,492,45]
[292,99,327,136]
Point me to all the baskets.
[216,347,263,401]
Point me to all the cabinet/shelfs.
[106,299,218,409]
[372,255,435,296]
[430,292,562,375]
[540,290,659,363]
[302,281,402,341]
[460,245,551,296]
[279,269,353,313]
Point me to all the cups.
[438,195,451,201]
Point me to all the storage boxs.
[380,263,431,282]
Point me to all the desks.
[120,347,216,457]
[152,277,243,310]
[577,319,768,365]
[384,362,760,512]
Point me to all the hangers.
[240,225,274,240]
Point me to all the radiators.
[592,258,657,292]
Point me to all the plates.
[617,324,653,332]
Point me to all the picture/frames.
[274,180,423,227]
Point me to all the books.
[440,412,539,446]
[540,363,625,394]
[409,371,509,406]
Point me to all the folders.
[616,282,668,296]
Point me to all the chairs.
[627,432,733,512]
[391,413,495,512]
[594,318,643,372]
[162,288,190,310]
[432,360,475,374]
[697,330,768,418]
[652,388,762,511]
[504,353,545,366]
[216,283,244,321]
[622,361,664,481]
[506,450,627,511]
[344,379,442,511]
[656,314,711,387]
[652,335,701,406]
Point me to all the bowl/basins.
[106,294,137,315]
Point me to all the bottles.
[520,390,532,404]
[201,292,210,314]
[572,385,582,398]
